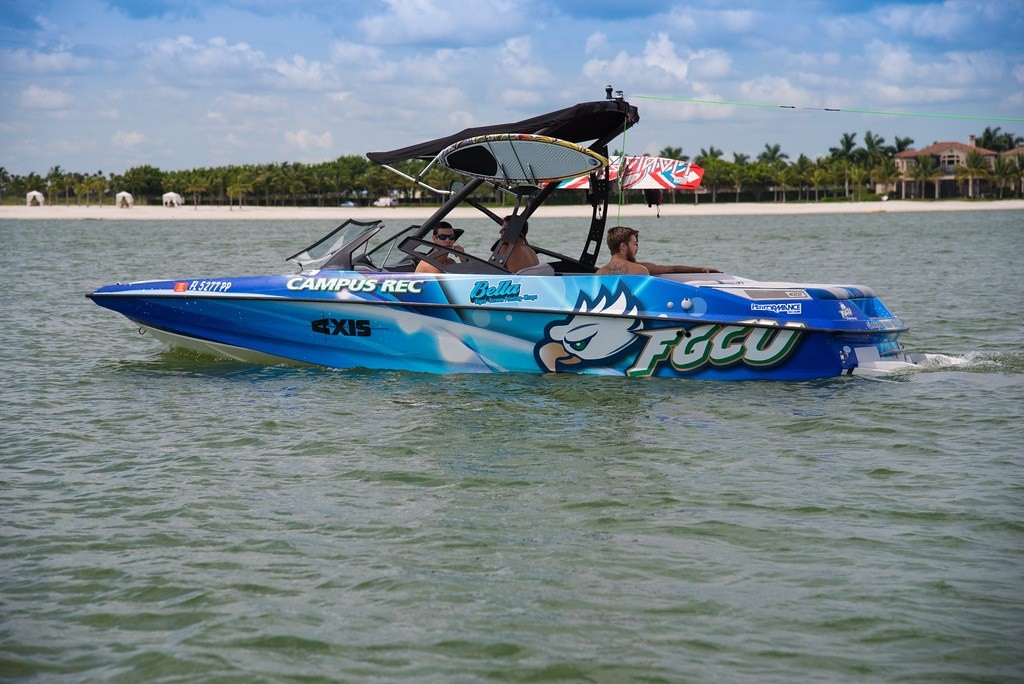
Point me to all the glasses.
[502,226,508,231]
[434,233,455,241]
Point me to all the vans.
[372,195,400,207]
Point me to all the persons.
[415,222,467,273]
[595,226,649,274]
[499,214,539,272]
[625,228,721,274]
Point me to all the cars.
[341,200,357,207]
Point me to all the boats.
[85,84,941,382]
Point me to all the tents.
[26,190,44,207]
[163,192,182,208]
[116,191,133,209]
